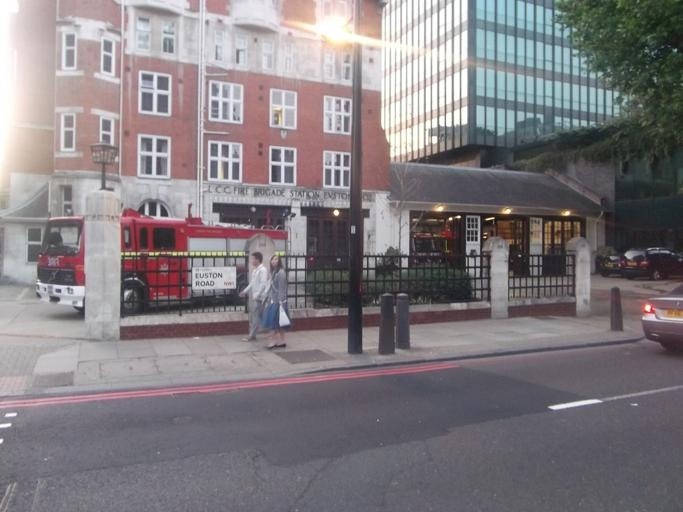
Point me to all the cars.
[600,254,625,277]
[621,247,683,281]
[641,284,683,354]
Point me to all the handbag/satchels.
[278,301,292,327]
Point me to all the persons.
[238,252,271,342]
[261,254,292,350]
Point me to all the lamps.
[91,139,120,190]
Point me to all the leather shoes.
[240,334,286,350]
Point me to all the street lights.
[321,10,363,353]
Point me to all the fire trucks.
[35,203,292,314]
